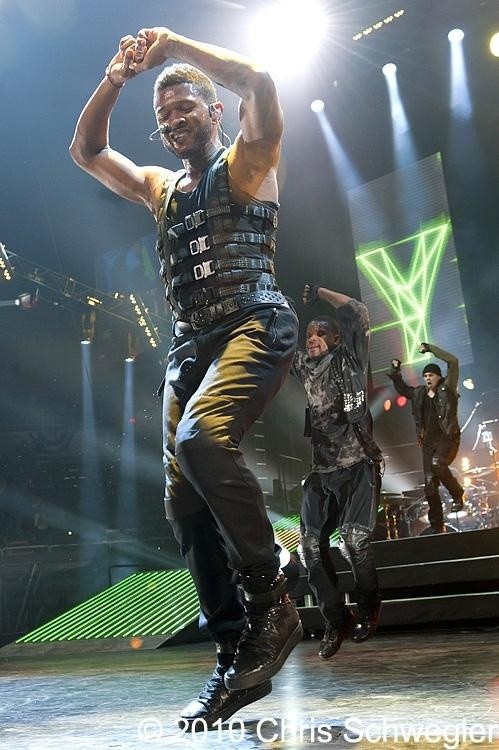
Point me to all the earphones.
[208,105,221,122]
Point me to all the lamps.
[0,243,161,364]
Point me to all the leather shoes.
[318,614,353,658]
[351,605,382,642]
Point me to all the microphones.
[148,121,172,143]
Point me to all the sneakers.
[420,526,445,535]
[452,497,462,511]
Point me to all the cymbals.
[380,490,401,496]
[462,467,496,478]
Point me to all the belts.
[174,290,290,336]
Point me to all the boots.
[223,573,303,690]
[178,639,271,731]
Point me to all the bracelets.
[105,66,125,88]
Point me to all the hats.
[423,364,441,376]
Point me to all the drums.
[447,500,472,519]
[418,501,446,524]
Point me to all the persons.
[386,344,464,535]
[288,283,386,660]
[69,27,300,732]
